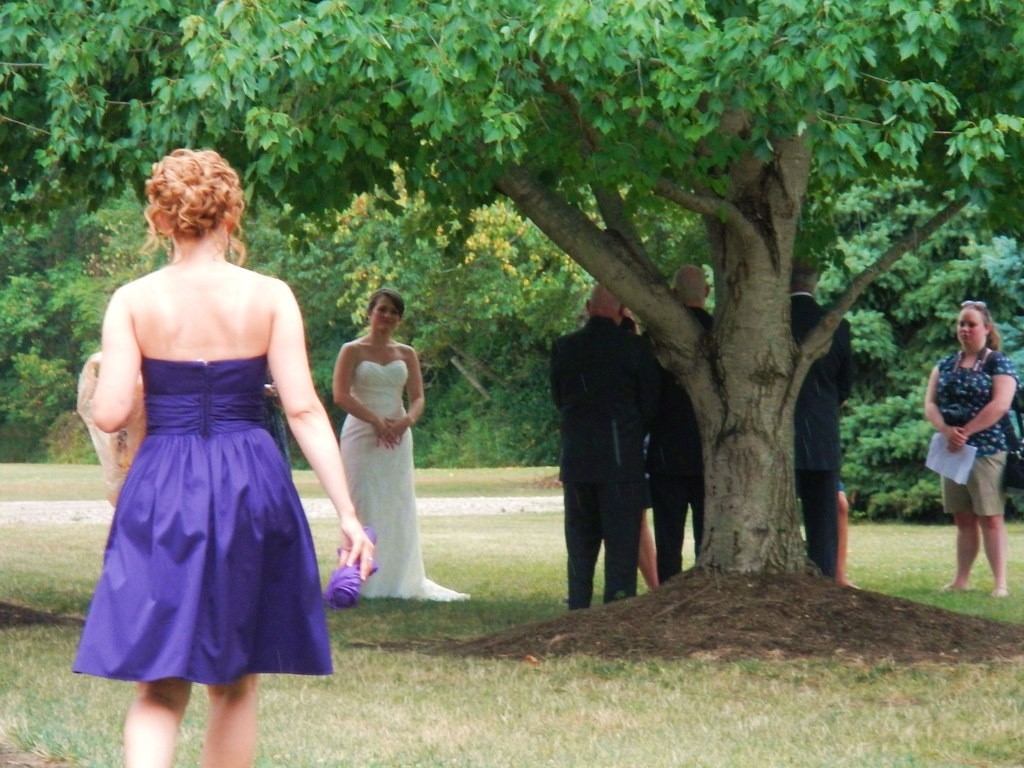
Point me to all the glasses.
[960,300,986,309]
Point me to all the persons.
[73,147,378,768]
[637,264,721,582]
[789,255,854,582]
[548,282,662,613]
[924,301,1020,598]
[618,303,659,590]
[837,478,864,590]
[330,290,472,603]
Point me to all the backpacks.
[988,351,1024,493]
